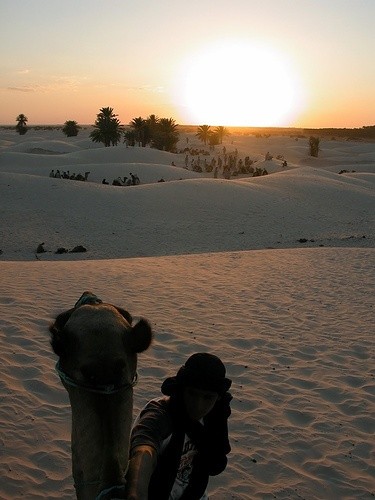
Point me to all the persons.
[122,353,233,500]
[47,291,157,500]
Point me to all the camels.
[48,291,153,500]
[74,172,90,181]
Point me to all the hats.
[158,352,233,396]
